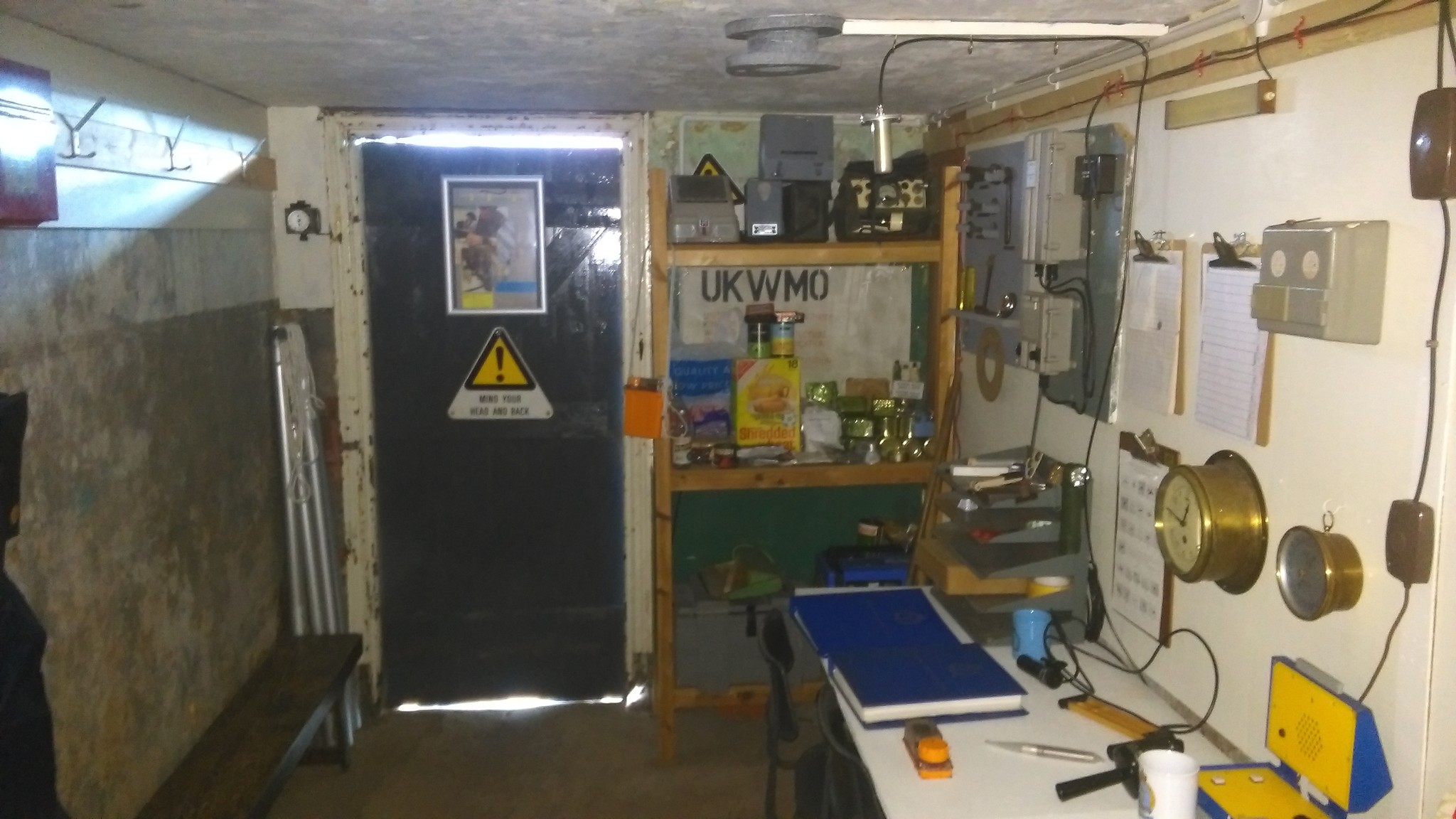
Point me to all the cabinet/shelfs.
[649,167,961,766]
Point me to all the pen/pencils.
[993,737,1100,762]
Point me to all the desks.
[793,585,1233,819]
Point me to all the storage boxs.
[731,358,801,453]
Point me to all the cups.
[1012,609,1052,661]
[1137,749,1201,819]
[688,444,738,469]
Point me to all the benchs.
[134,632,364,819]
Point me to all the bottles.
[671,409,692,470]
